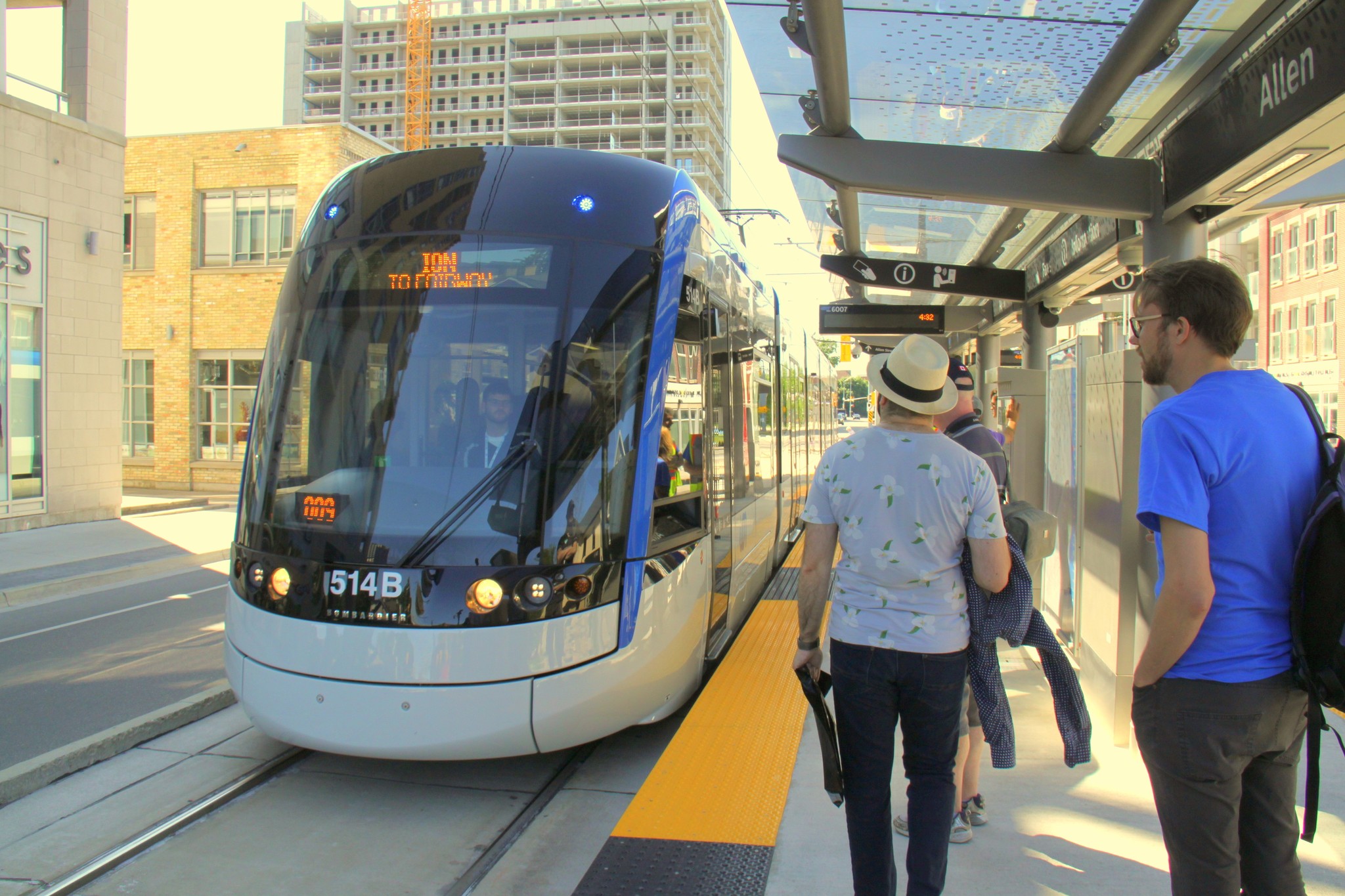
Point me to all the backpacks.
[1280,381,1345,714]
[1000,446,1057,577]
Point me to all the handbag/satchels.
[794,663,843,807]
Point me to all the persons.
[789,332,1014,896]
[657,405,683,497]
[680,433,703,494]
[1115,258,1337,896]
[650,446,672,500]
[455,379,544,469]
[893,355,1018,842]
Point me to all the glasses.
[487,399,511,407]
[990,402,997,406]
[1130,314,1169,337]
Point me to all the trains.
[220,142,845,765]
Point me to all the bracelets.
[797,636,820,650]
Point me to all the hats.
[866,333,958,414]
[972,396,984,419]
[947,357,974,391]
[568,500,575,509]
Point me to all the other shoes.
[961,793,988,826]
[893,810,972,843]
